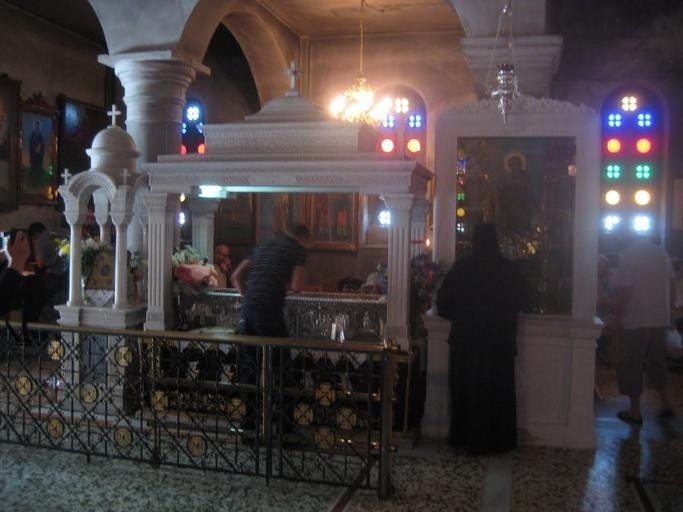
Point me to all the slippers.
[617,409,642,424]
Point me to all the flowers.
[55,236,99,276]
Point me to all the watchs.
[228,215,314,430]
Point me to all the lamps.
[326,0,392,127]
[485,1,520,123]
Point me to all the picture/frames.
[0,72,115,215]
[269,190,360,253]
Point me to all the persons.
[435,220,531,455]
[25,119,45,190]
[0,222,237,323]
[498,155,536,231]
[612,222,682,426]
